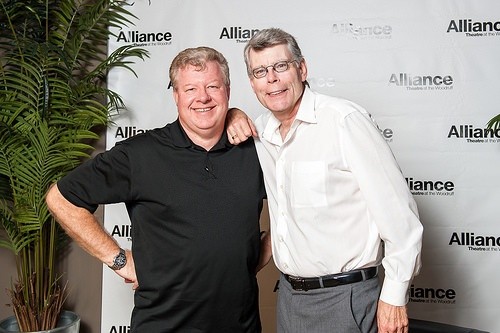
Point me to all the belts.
[281,266,378,291]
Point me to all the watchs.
[108,248,127,270]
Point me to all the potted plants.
[0,0,152,333]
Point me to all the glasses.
[249,59,299,79]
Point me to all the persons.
[224,28,424,333]
[46,46,272,333]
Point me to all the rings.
[232,134,238,139]
